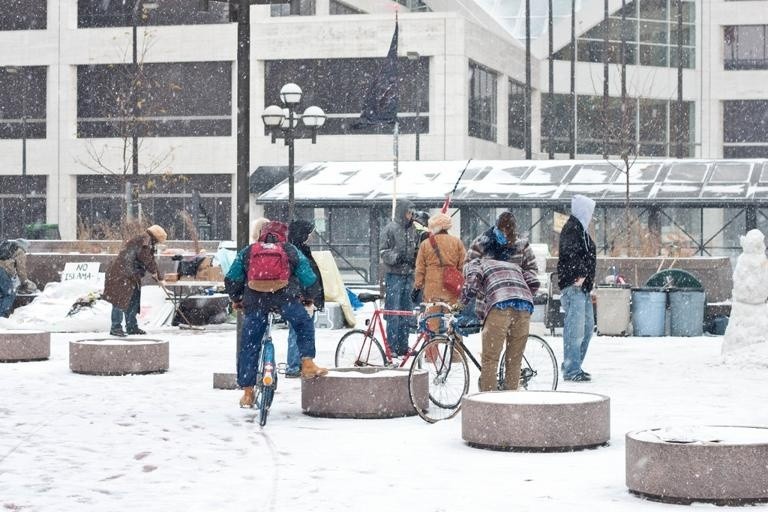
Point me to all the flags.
[342,19,399,130]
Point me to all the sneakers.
[128,325,146,334]
[110,328,124,336]
[564,372,592,381]
[398,347,417,356]
[390,351,398,358]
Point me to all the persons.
[409,212,431,336]
[103,223,167,338]
[377,197,434,360]
[221,221,328,409]
[1,234,39,318]
[463,212,541,289]
[283,219,324,380]
[557,195,596,381]
[415,209,466,365]
[460,257,537,392]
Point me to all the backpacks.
[247,232,291,292]
[1,240,17,260]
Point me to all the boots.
[240,386,254,405]
[301,357,328,379]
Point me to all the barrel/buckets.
[595,283,632,337]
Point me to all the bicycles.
[335,292,467,410]
[236,299,312,428]
[407,313,558,424]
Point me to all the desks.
[160,277,231,328]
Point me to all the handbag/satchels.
[444,266,464,293]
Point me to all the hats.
[147,225,167,243]
[17,238,28,248]
[260,221,288,241]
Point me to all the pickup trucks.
[21,223,62,240]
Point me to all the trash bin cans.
[595,284,704,337]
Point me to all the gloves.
[411,288,420,303]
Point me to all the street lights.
[407,52,421,161]
[5,64,27,225]
[131,0,160,226]
[262,82,326,225]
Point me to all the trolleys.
[545,272,597,336]
